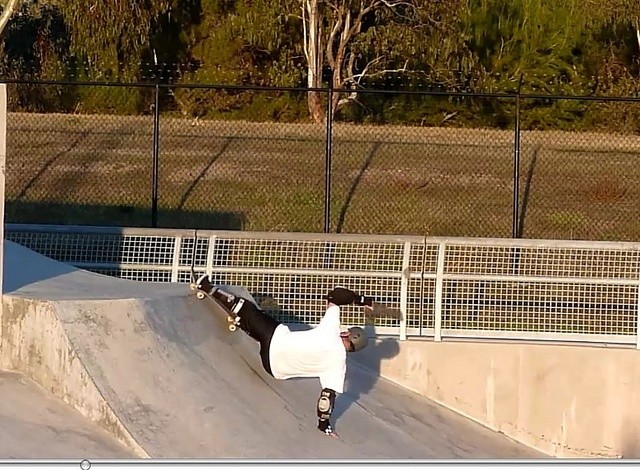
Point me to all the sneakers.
[196,274,212,290]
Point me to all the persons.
[196,274,374,439]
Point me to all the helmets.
[349,327,368,352]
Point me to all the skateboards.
[190,266,241,331]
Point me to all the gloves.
[318,419,334,435]
[361,296,372,310]
